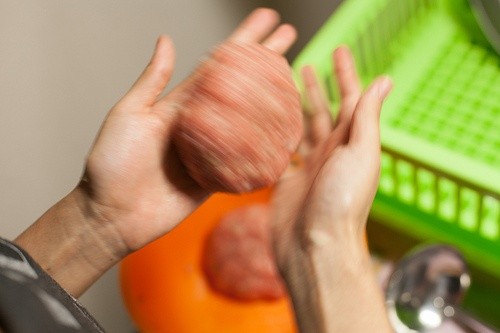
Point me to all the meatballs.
[203,204,286,299]
[174,39,305,193]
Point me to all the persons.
[0,7,398,333]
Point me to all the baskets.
[287,2,498,270]
